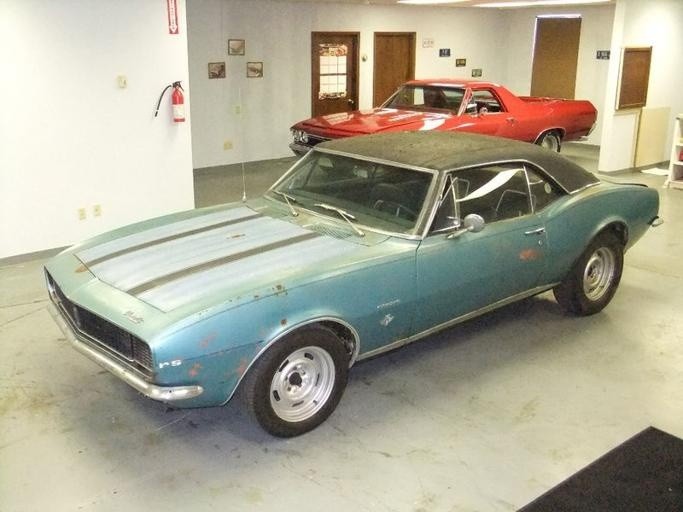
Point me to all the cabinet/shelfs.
[660,112,682,194]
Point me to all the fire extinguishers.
[155,80,186,123]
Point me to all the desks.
[515,426,682,512]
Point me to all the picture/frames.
[245,61,264,79]
[207,62,225,78]
[227,38,245,56]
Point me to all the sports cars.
[38,127,661,446]
[282,74,601,175]
